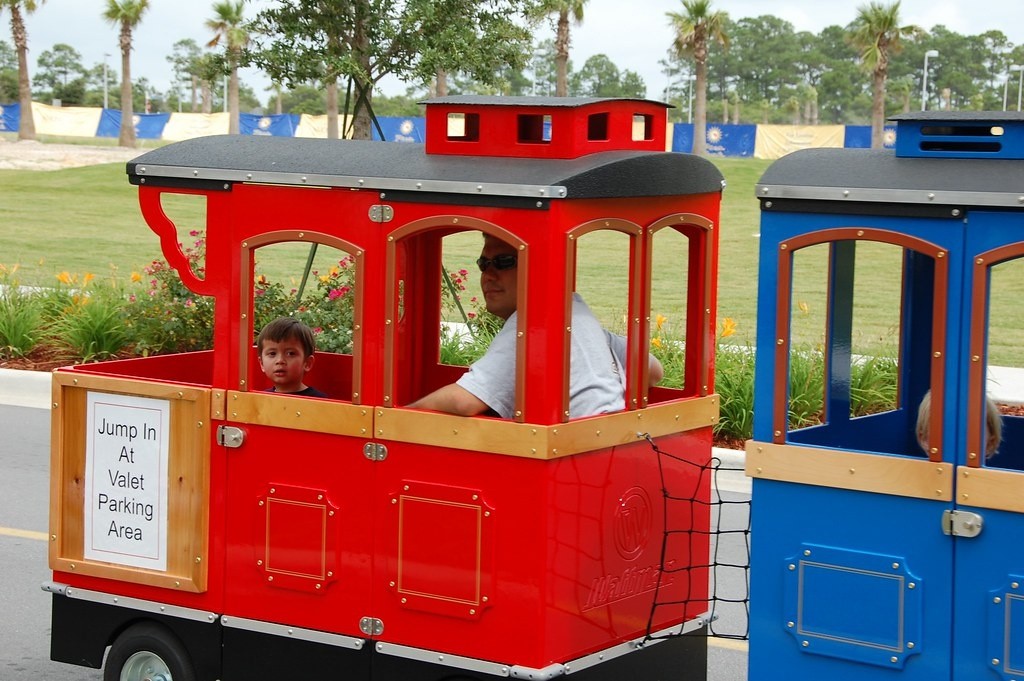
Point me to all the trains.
[46,93,1024,681]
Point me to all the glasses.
[476,254,518,272]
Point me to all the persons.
[255,319,330,399]
[916,385,1004,458]
[405,229,663,421]
